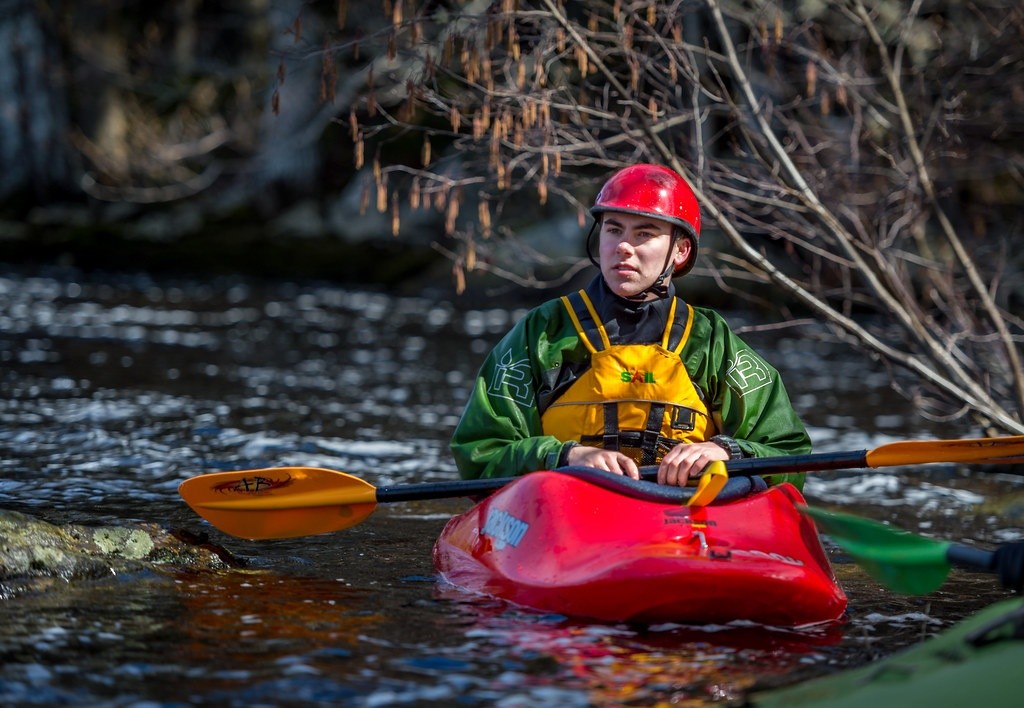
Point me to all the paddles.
[179,434,1024,543]
[793,503,1022,599]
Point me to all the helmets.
[590,164,700,278]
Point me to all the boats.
[430,463,848,633]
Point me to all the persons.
[449,164,814,502]
